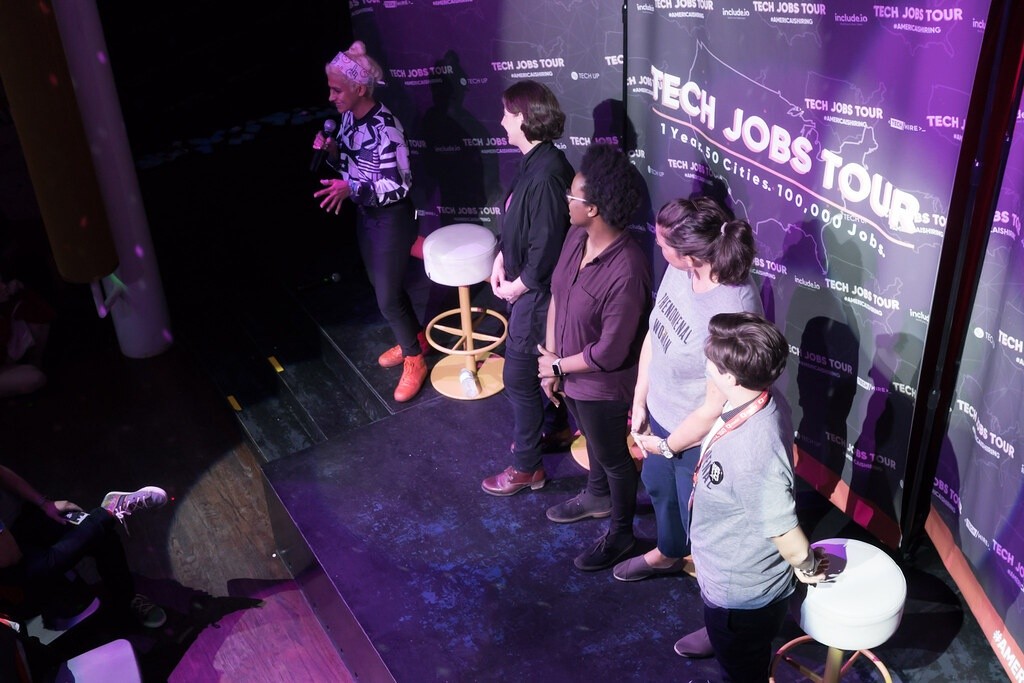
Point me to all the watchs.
[551,358,566,376]
[661,439,673,459]
[353,181,362,197]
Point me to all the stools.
[768,537,907,683]
[423,222,505,401]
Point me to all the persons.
[0,464,167,627]
[312,40,431,402]
[0,255,57,397]
[538,151,652,570]
[687,311,830,683]
[614,199,764,658]
[483,81,576,496]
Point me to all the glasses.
[566,190,588,204]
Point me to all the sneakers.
[100,485,167,539]
[545,490,611,522]
[392,354,429,402]
[378,332,434,367]
[103,592,167,629]
[574,524,636,571]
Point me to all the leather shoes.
[481,464,545,496]
[510,424,572,455]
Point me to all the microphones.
[310,119,337,172]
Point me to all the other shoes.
[674,626,714,658]
[613,549,685,582]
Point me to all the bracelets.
[800,568,815,575]
[657,439,664,455]
[0,520,6,534]
[38,496,46,507]
